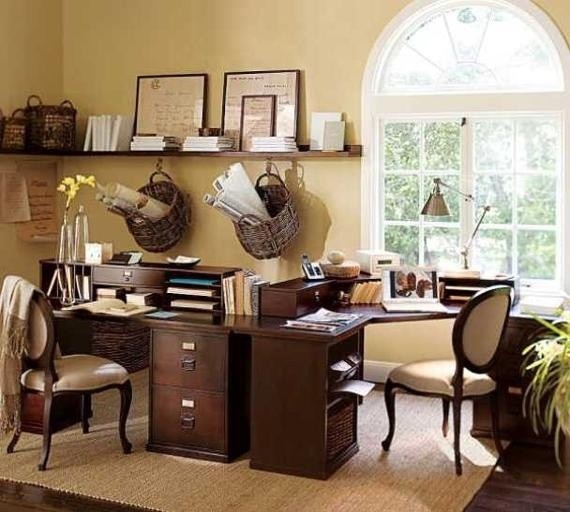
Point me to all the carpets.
[0,364,515,512]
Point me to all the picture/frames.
[130,67,302,151]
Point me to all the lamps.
[419,176,492,270]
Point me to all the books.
[309,111,342,151]
[80,111,300,154]
[167,287,217,297]
[323,120,345,151]
[61,285,157,318]
[222,269,271,316]
[381,297,448,313]
[348,279,382,303]
[171,299,219,310]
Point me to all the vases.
[58,213,78,265]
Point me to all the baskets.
[232,173,302,260]
[0,94,76,153]
[51,319,150,374]
[125,171,192,253]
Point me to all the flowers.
[56,172,98,222]
